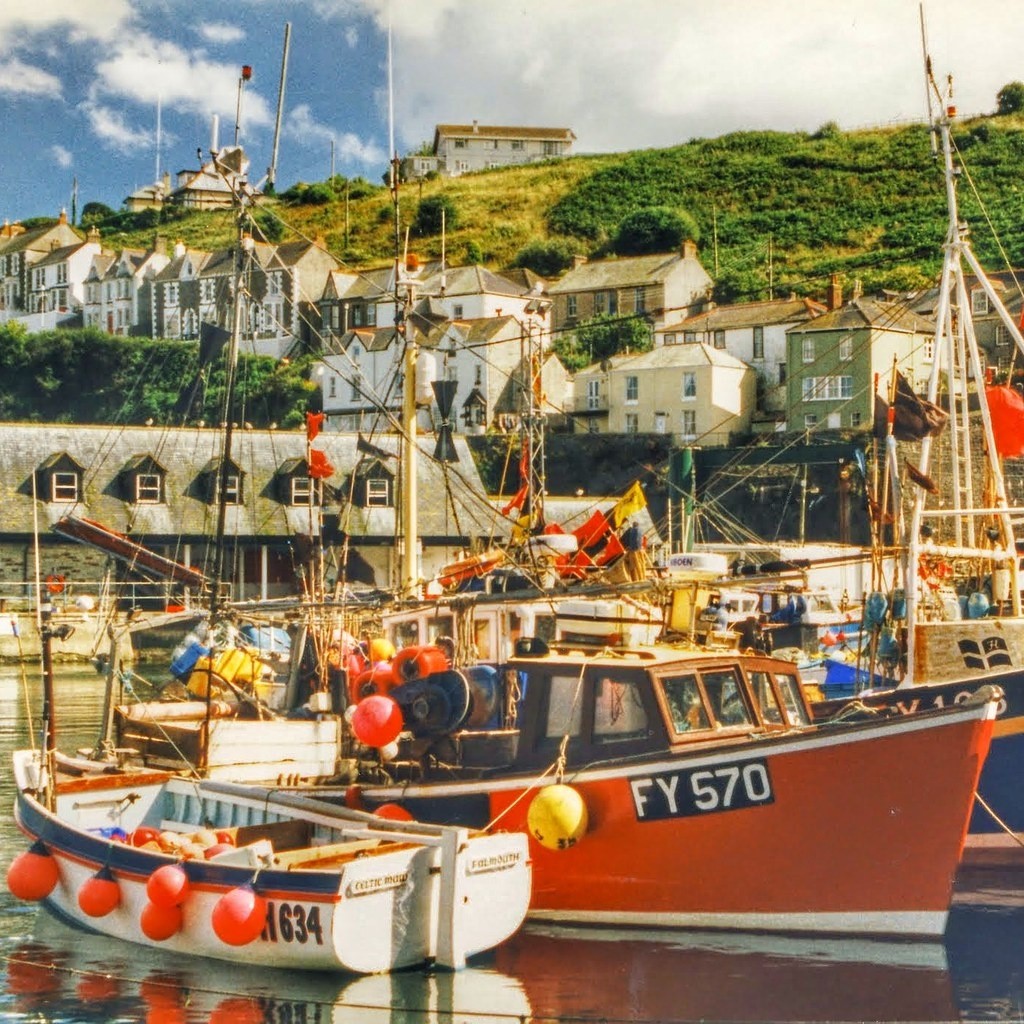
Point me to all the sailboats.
[2,0,1024,1022]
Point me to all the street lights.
[231,63,253,206]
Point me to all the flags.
[875,373,929,442]
[608,483,661,548]
[307,413,324,441]
[310,450,333,478]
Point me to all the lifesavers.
[45,573,65,593]
